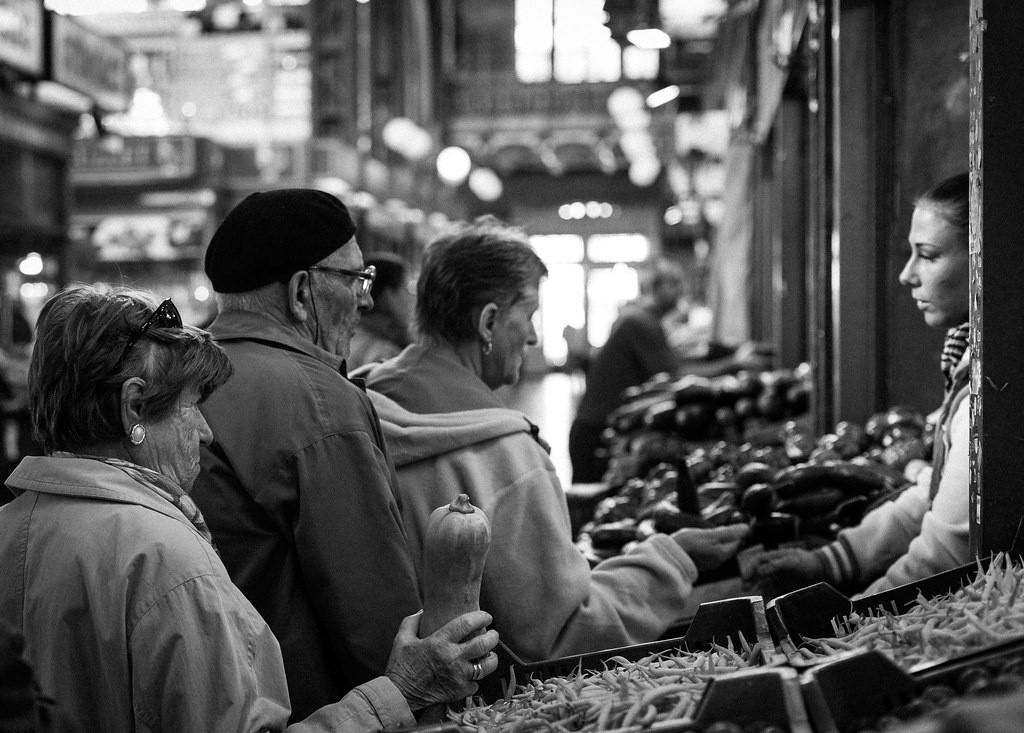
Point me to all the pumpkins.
[418,494,490,662]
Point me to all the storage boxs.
[456,555,1024,731]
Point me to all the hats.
[204,188,358,294]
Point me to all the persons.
[191,188,448,726]
[348,230,754,704]
[568,251,762,483]
[344,251,417,374]
[742,169,968,600]
[0,283,499,732]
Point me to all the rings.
[472,661,482,682]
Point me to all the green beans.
[796,548,1023,675]
[438,630,763,733]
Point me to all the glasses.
[307,265,377,301]
[103,297,184,380]
